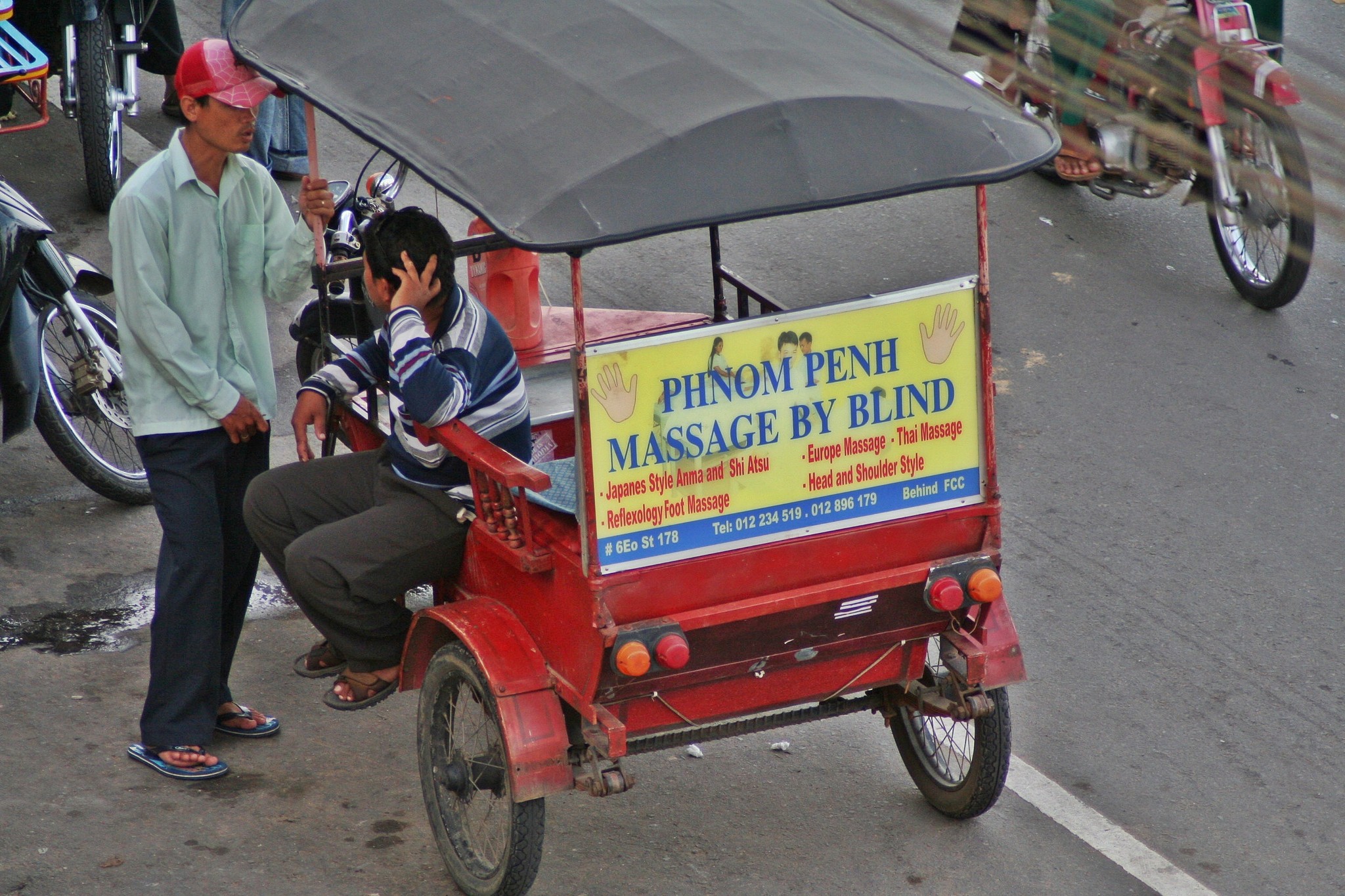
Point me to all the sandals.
[322,666,400,711]
[293,638,348,679]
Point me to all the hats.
[173,36,286,110]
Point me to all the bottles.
[467,216,544,350]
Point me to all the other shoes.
[161,90,184,117]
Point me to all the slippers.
[980,65,1022,107]
[126,743,229,780]
[206,701,280,738]
[1226,130,1255,159]
[1053,141,1104,182]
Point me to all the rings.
[241,434,249,439]
[321,200,325,207]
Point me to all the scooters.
[0,178,168,504]
[45,0,162,213]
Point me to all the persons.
[0,0,185,123]
[241,203,532,711]
[949,0,1284,182]
[106,38,335,779]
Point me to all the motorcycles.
[219,0,1064,896]
[960,1,1316,311]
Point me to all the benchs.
[356,360,574,426]
[413,417,578,549]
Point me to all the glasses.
[372,205,425,288]
